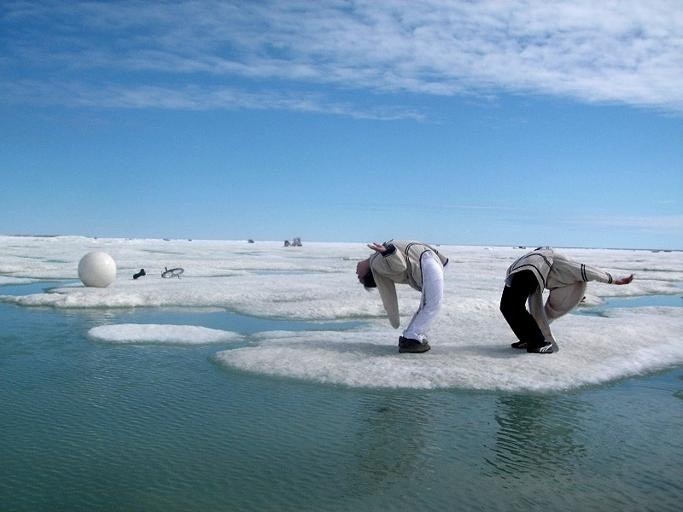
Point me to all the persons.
[355,238,449,353]
[499,249,634,353]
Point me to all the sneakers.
[526,341,553,354]
[512,341,527,349]
[398,336,430,354]
